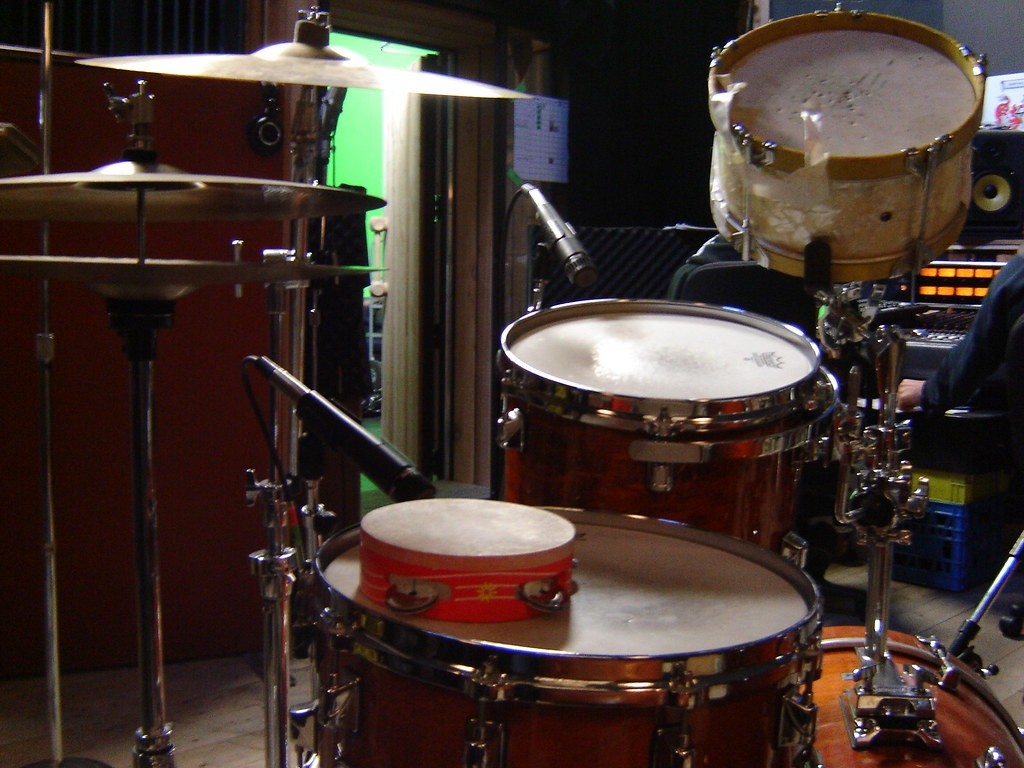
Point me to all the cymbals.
[0,159,387,221]
[70,42,532,100]
[383,576,579,620]
[0,254,387,302]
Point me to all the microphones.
[519,183,599,290]
[253,355,436,503]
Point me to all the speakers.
[959,129,1024,237]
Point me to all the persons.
[892,253,1024,641]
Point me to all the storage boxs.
[835,493,1005,592]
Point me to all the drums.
[358,497,583,625]
[303,495,823,768]
[494,295,822,570]
[702,5,985,287]
[810,625,1023,768]
